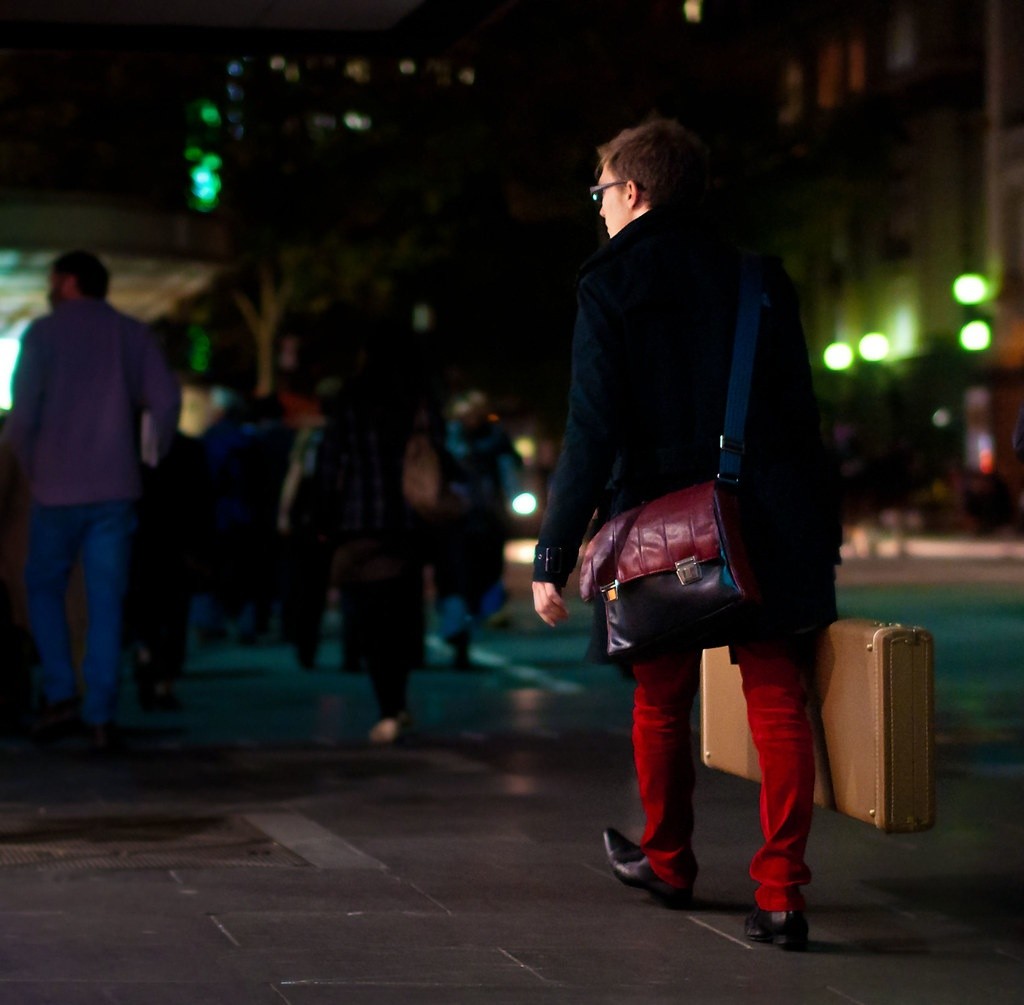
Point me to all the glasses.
[590,183,644,205]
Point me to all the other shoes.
[368,711,412,749]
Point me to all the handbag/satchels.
[580,478,761,654]
[402,399,471,513]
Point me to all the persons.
[1,251,522,754]
[533,121,841,948]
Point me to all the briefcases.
[699,616,936,835]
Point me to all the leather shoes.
[601,827,694,910]
[745,904,809,953]
